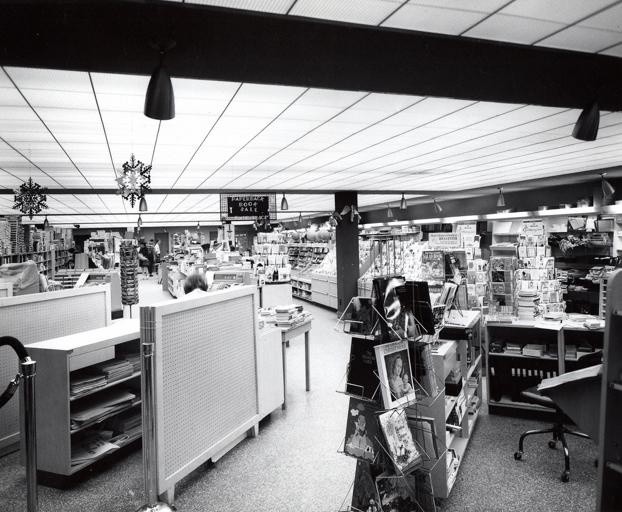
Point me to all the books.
[68,353,141,465]
[342,279,438,512]
[259,306,309,329]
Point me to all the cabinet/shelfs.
[0,213,622,512]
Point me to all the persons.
[181,274,209,298]
[65,248,80,266]
[135,237,162,280]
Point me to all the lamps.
[139,196,149,212]
[596,172,617,199]
[400,194,409,210]
[280,192,289,211]
[136,214,143,226]
[431,197,445,213]
[298,212,303,223]
[307,215,313,226]
[385,205,394,219]
[44,216,50,228]
[193,214,274,229]
[142,42,177,120]
[493,186,507,208]
[572,75,610,141]
[325,204,364,229]
